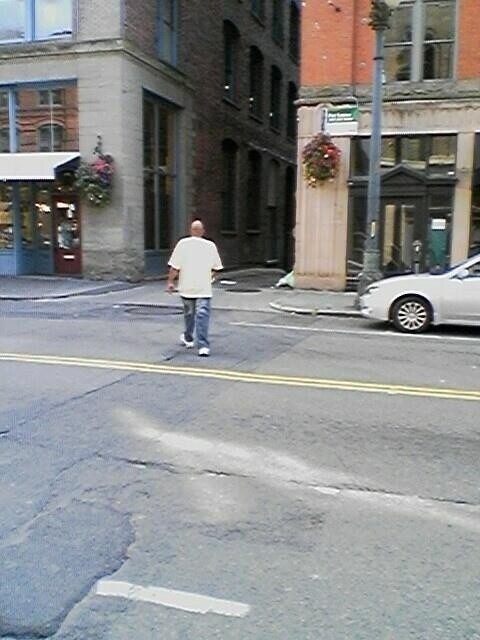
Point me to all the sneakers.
[180,333,194,349]
[198,347,210,356]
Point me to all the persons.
[167,220,224,356]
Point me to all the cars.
[354,251,480,335]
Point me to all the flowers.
[73,134,116,208]
[301,131,343,189]
